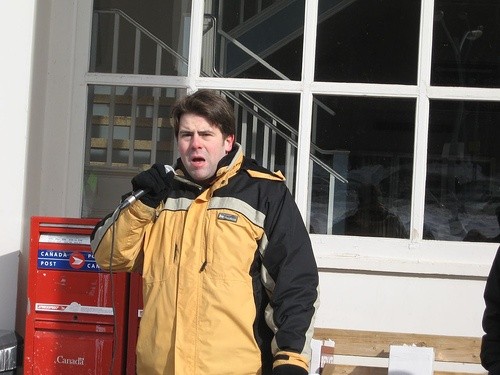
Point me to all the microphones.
[119,165,175,211]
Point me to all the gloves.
[131,163,175,209]
[273,364,308,375]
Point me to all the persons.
[90,89,320,375]
[344,184,407,239]
[480,245,500,375]
